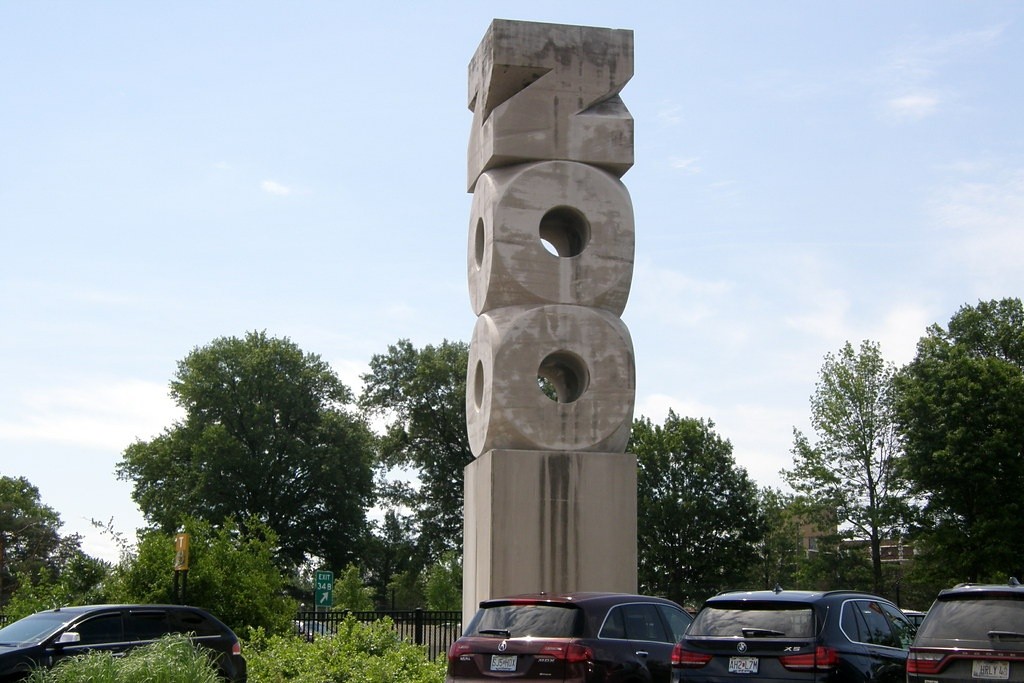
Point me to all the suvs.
[444,591,696,682]
[905,581,1024,682]
[0,602,248,683]
[671,584,912,683]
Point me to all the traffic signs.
[315,571,333,609]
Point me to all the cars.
[864,608,926,634]
[292,620,337,643]
